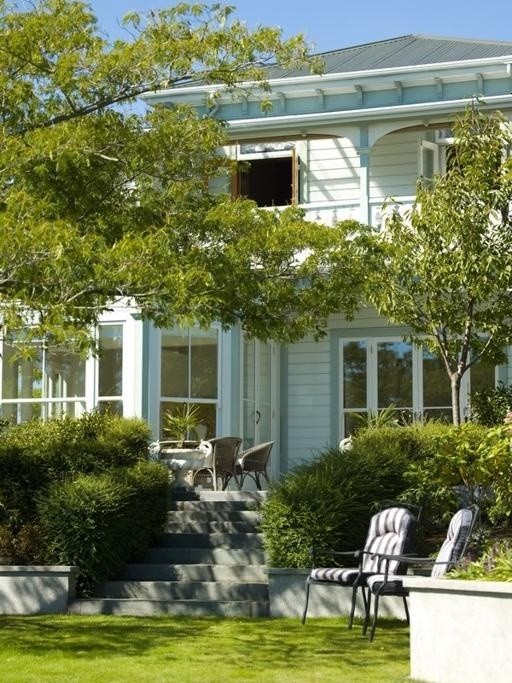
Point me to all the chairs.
[192,435,274,491]
[301,502,480,641]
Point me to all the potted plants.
[158,404,202,501]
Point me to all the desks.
[159,438,198,447]
